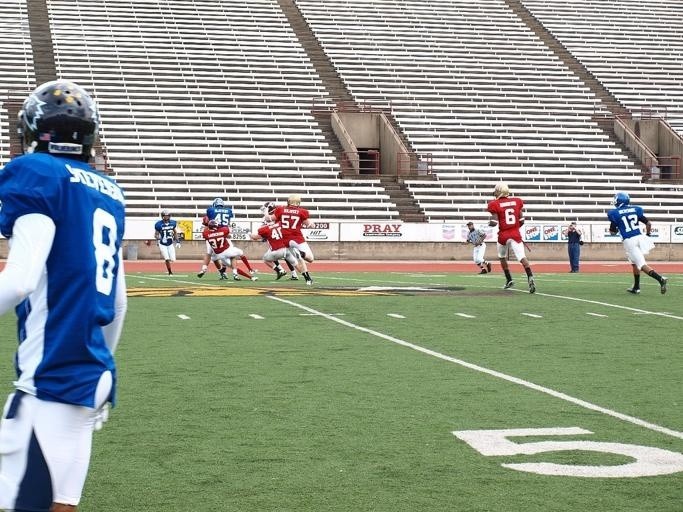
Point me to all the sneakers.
[503,280,515,290]
[233,273,242,280]
[275,269,287,281]
[569,270,579,273]
[527,276,536,295]
[197,270,205,279]
[478,269,487,274]
[292,248,301,260]
[289,275,298,280]
[219,272,229,280]
[306,279,313,285]
[169,271,174,278]
[625,284,642,294]
[487,262,491,273]
[660,275,668,295]
[250,276,259,282]
[249,269,258,275]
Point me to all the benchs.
[0,0,682,223]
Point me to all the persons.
[488,182,535,294]
[607,193,668,294]
[0,81,128,512]
[155,210,181,276]
[197,194,314,286]
[564,222,582,273]
[467,222,491,274]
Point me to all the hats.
[466,221,474,225]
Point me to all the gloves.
[93,402,110,432]
[175,241,181,248]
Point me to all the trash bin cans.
[127,244,138,260]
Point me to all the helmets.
[494,181,510,198]
[286,193,301,207]
[261,201,277,214]
[208,219,219,229]
[15,78,98,165]
[160,209,171,219]
[261,215,267,224]
[613,191,630,207]
[212,197,225,208]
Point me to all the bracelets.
[175,240,180,243]
[646,233,651,236]
